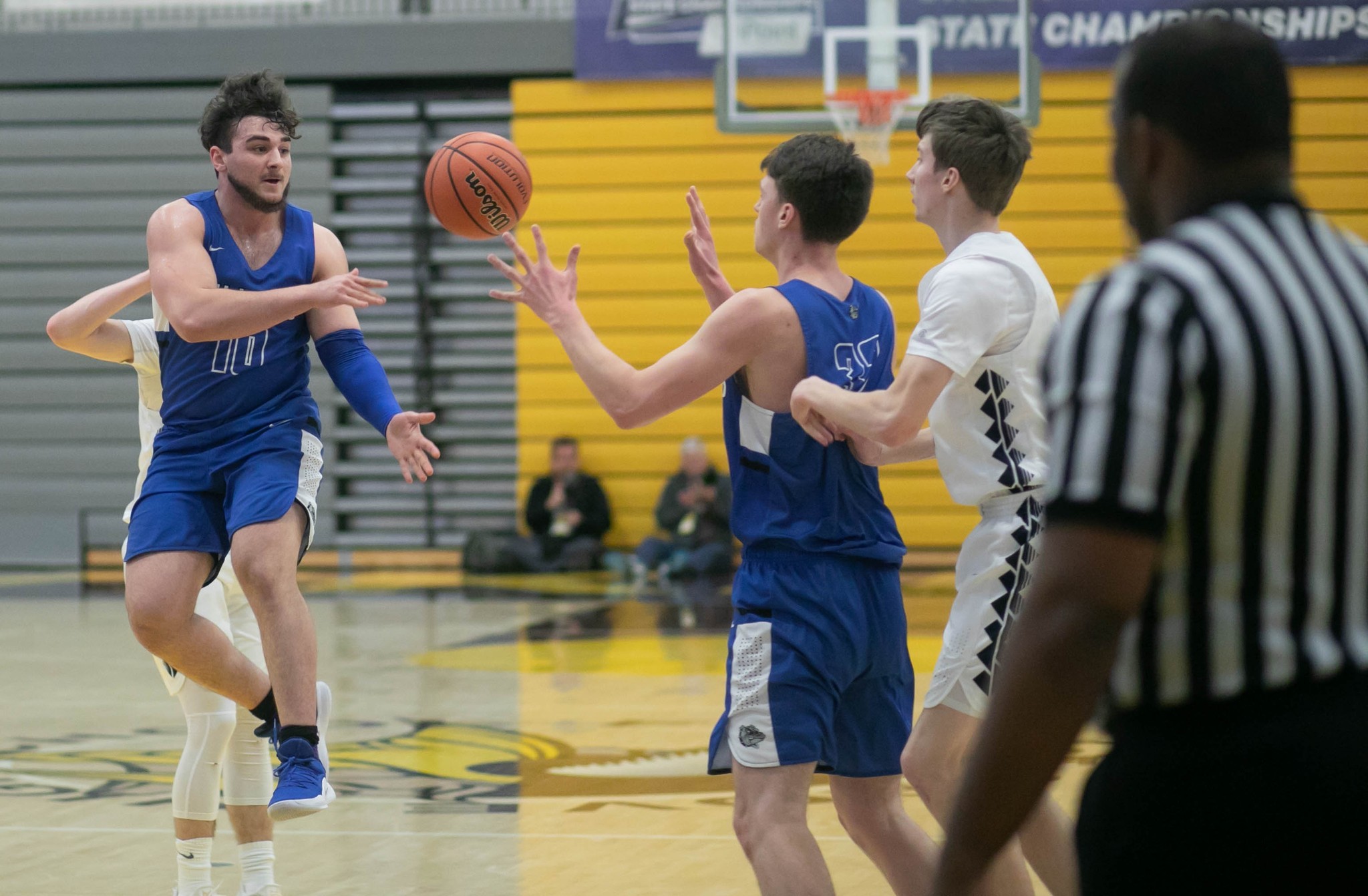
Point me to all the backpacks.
[464,529,540,574]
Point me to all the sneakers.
[269,740,336,822]
[251,681,331,781]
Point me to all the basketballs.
[423,130,534,242]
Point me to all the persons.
[515,436,611,574]
[924,11,1368,896]
[636,435,735,578]
[45,268,281,895]
[789,97,1083,896]
[485,131,941,895]
[122,67,441,823]
